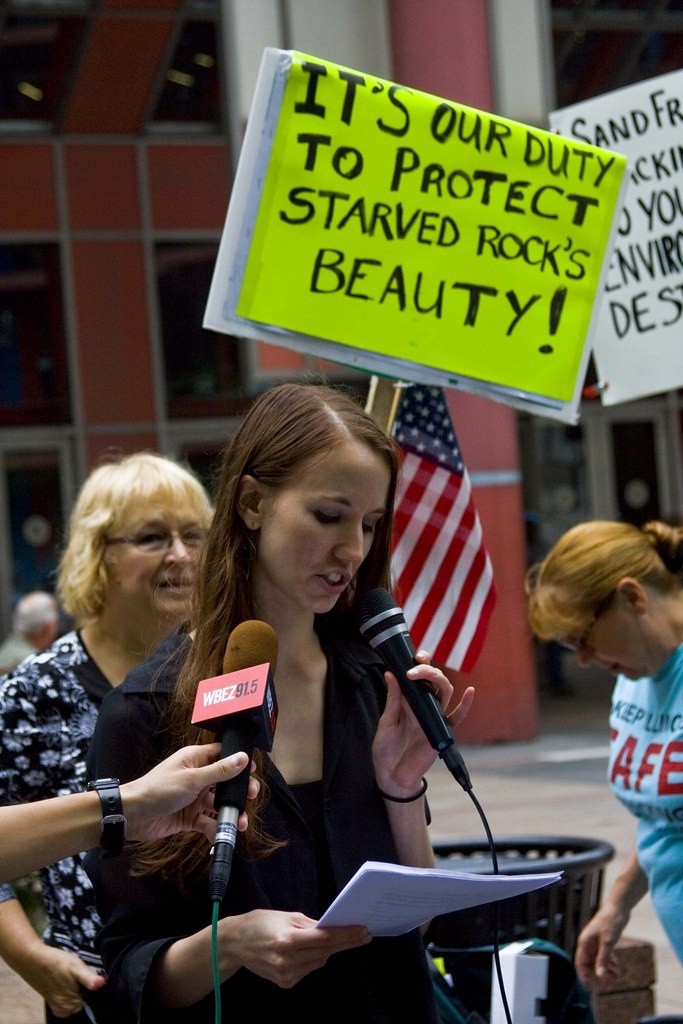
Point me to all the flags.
[386,378,497,679]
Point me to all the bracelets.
[375,778,428,804]
[88,778,129,851]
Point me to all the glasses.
[559,591,610,656]
[96,521,210,563]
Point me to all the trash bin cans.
[431,834,616,1023]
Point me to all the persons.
[89,381,472,1024]
[0,743,261,882]
[525,519,683,989]
[0,453,218,1024]
[0,591,60,677]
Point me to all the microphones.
[190,621,279,898]
[354,586,474,792]
